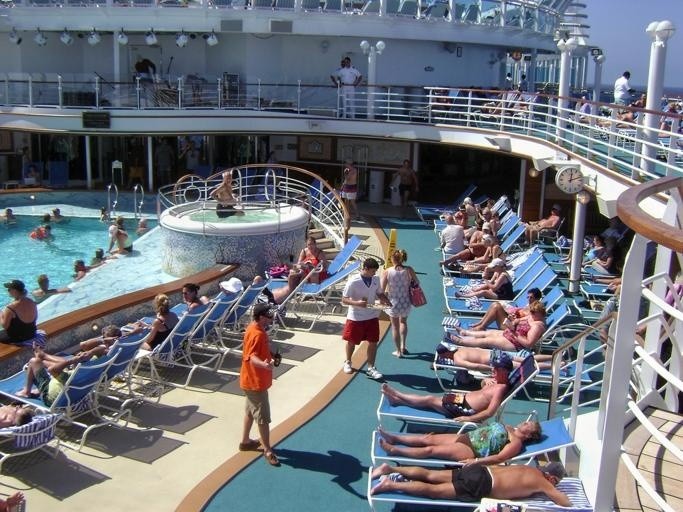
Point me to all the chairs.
[0,157,362,466]
[408,86,683,169]
[377,354,540,434]
[370,416,577,467]
[368,465,594,511]
[415,182,661,402]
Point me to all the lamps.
[7,29,218,47]
[528,167,539,178]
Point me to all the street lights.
[638,19,677,179]
[359,40,385,120]
[556,36,577,144]
[593,53,607,114]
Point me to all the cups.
[360,296,367,308]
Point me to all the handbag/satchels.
[409,286,427,307]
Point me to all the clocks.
[553,167,585,194]
[511,49,522,61]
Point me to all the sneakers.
[367,367,383,379]
[344,360,352,374]
[240,441,261,451]
[262,449,279,465]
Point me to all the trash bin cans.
[369,171,385,204]
[391,174,402,206]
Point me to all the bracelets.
[476,457,479,464]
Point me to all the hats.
[4,280,23,291]
[253,304,273,315]
[488,258,505,267]
[490,353,513,370]
[220,277,244,293]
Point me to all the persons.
[378,421,542,468]
[135,54,156,107]
[340,158,360,221]
[430,72,683,138]
[342,257,393,380]
[330,59,348,118]
[337,56,362,118]
[380,249,419,358]
[389,159,419,221]
[380,355,513,423]
[370,460,572,507]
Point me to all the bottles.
[273,346,280,366]
